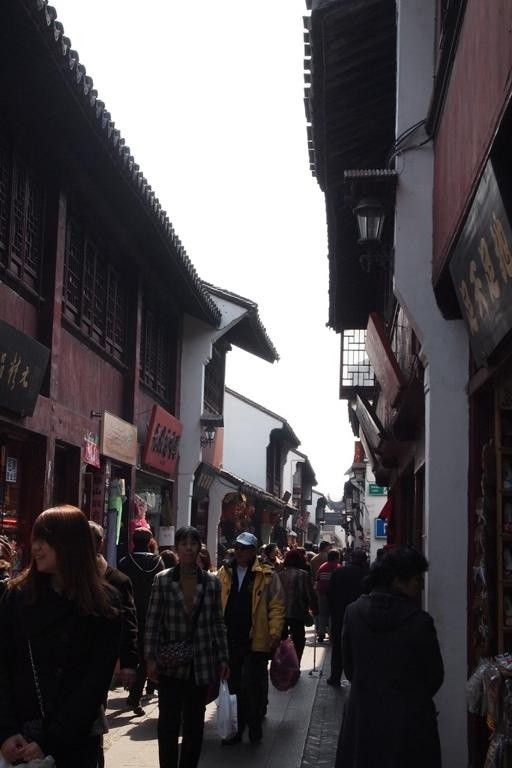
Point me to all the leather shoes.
[249,726,262,742]
[327,679,340,685]
[221,734,242,744]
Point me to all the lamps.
[199,424,217,448]
[351,195,390,250]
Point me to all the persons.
[89,519,179,717]
[1,503,118,768]
[141,525,226,768]
[215,531,286,746]
[259,539,386,689]
[334,545,444,768]
[198,543,236,578]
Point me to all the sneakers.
[127,700,146,715]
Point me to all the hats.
[235,531,258,547]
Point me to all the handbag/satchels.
[21,702,108,743]
[155,637,194,670]
